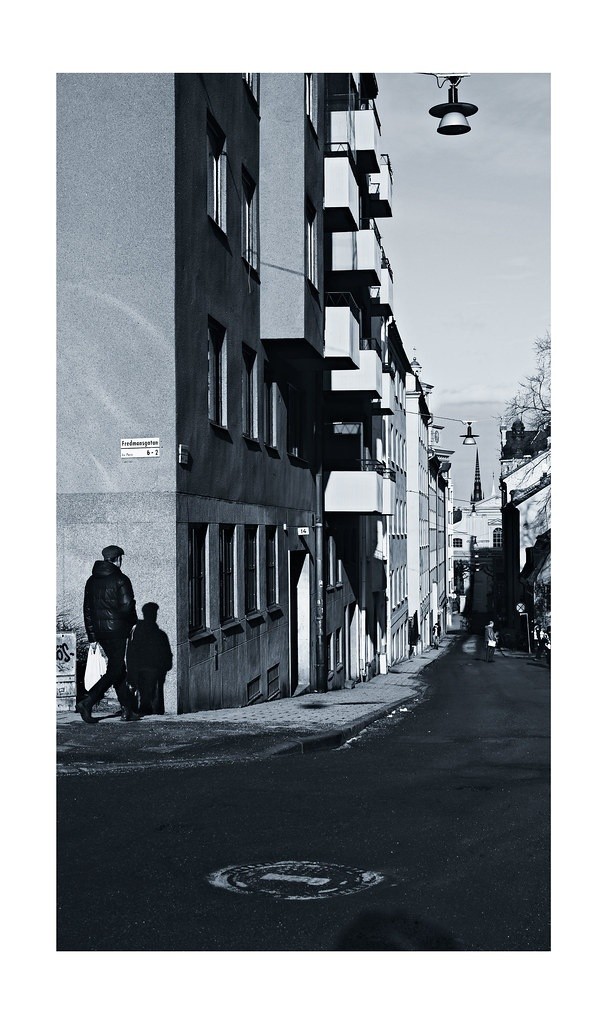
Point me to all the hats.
[102,545,125,558]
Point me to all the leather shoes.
[75,696,98,723]
[121,706,140,721]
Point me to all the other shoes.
[534,658,541,660]
[488,661,495,662]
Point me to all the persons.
[432,623,441,650]
[534,620,551,661]
[485,621,499,662]
[75,545,140,724]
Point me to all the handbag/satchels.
[488,640,496,647]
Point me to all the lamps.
[467,504,479,518]
[459,422,480,445]
[429,73,479,136]
